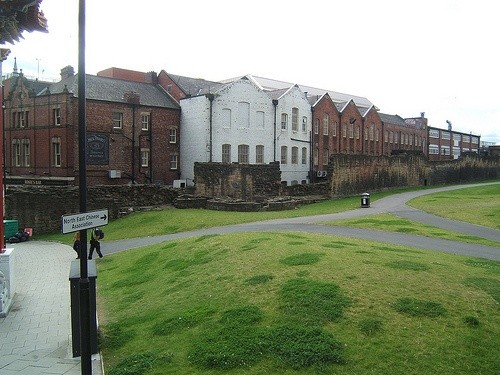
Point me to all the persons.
[72,231,80,260]
[88,228,103,260]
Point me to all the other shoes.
[76,257,80,259]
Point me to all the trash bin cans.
[360,192,370,208]
[3,219,18,240]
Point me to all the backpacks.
[98,230,104,239]
